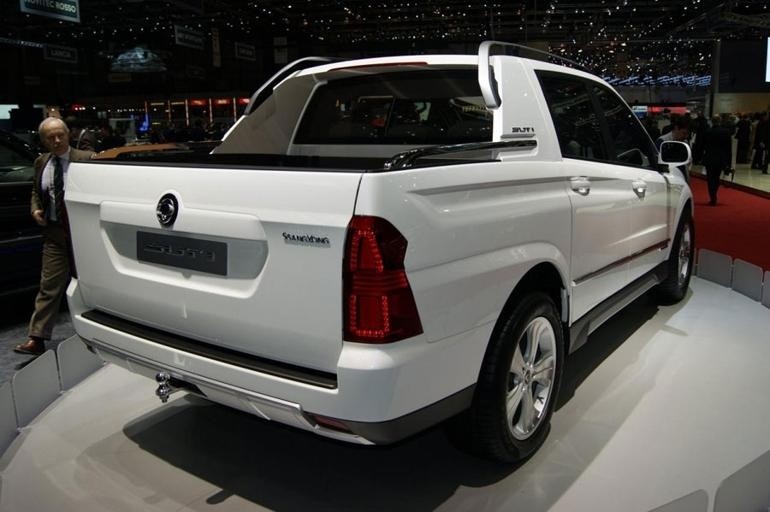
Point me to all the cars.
[0,127,76,321]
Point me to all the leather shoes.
[13,338,46,355]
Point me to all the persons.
[10,115,98,358]
[96,126,124,152]
[163,122,177,143]
[636,106,770,209]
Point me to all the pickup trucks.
[63,39,695,465]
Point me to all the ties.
[51,155,69,232]
